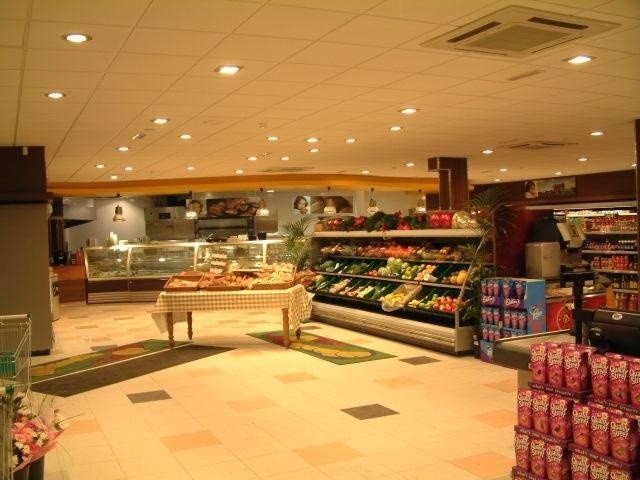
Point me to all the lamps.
[366,188,379,212]
[415,189,425,212]
[256,188,269,216]
[184,191,198,219]
[324,186,336,214]
[113,194,127,222]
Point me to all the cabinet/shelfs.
[83,237,310,305]
[296,228,496,356]
[566,201,640,313]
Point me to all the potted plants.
[454,183,520,359]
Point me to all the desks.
[160,284,303,348]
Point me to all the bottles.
[586,215,638,311]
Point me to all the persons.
[188,201,204,216]
[526,179,537,200]
[292,195,308,214]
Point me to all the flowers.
[1,388,87,473]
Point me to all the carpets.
[244,329,398,365]
[6,339,239,398]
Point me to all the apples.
[431,296,458,312]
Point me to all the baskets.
[164,274,203,291]
[252,266,301,290]
[207,274,247,290]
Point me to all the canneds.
[616,240,637,250]
